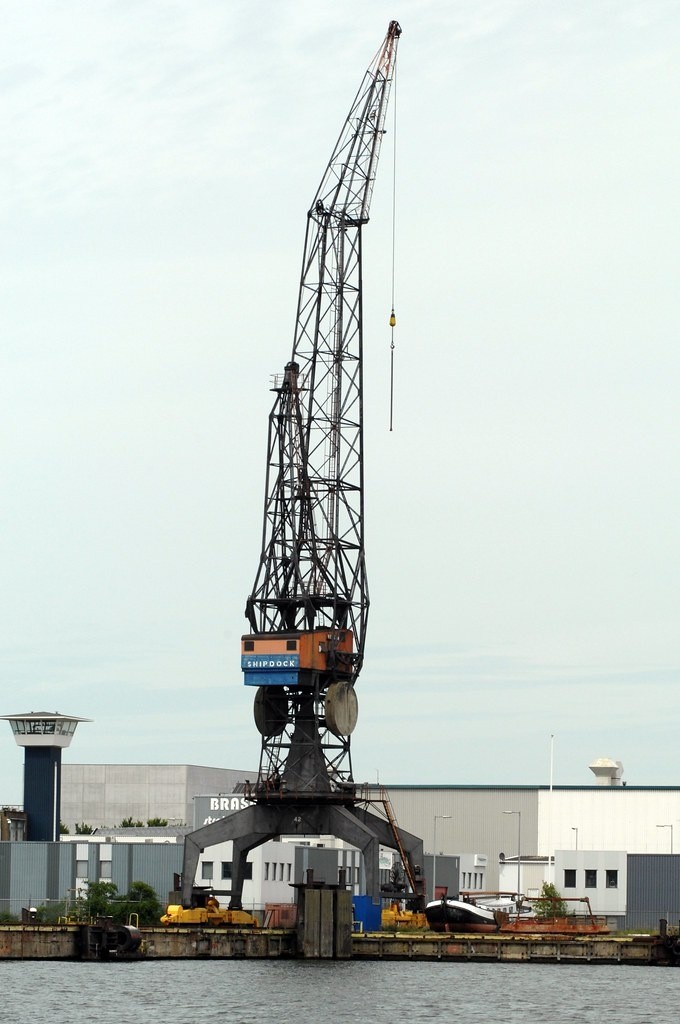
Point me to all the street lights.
[656,824,673,854]
[432,815,452,902]
[572,826,579,849]
[501,811,521,901]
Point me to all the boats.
[425,895,541,930]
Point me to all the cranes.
[181,18,425,920]
